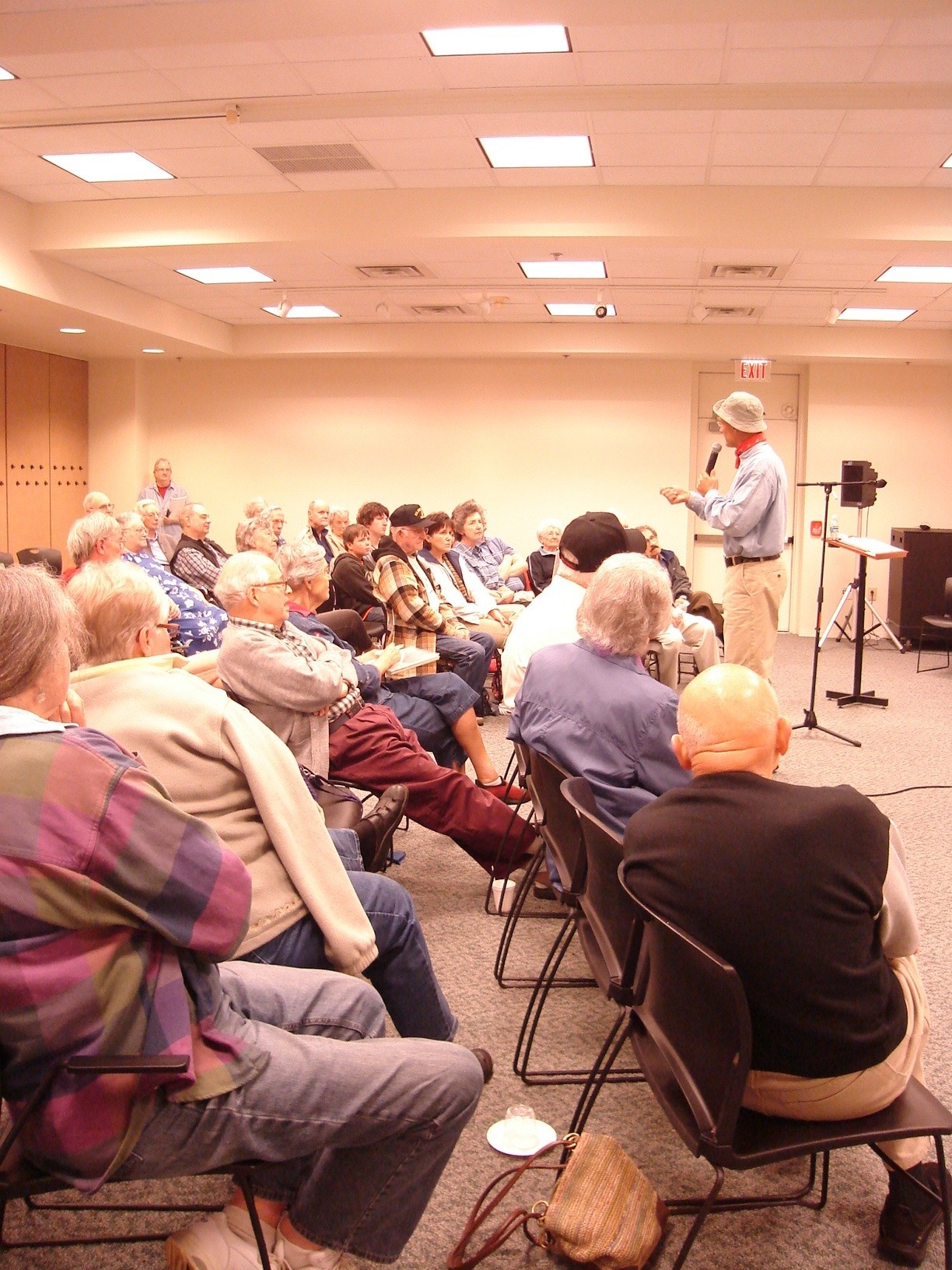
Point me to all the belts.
[329,696,366,735]
[725,554,780,567]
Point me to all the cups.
[502,1104,537,1151]
[492,879,516,912]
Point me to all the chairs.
[0,569,952,1270]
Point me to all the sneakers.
[165,1211,343,1270]
[874,1161,952,1268]
[475,776,532,805]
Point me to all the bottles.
[830,513,839,540]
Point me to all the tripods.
[818,509,907,655]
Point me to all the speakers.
[791,477,886,746]
[887,527,952,641]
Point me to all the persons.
[83,491,115,517]
[636,525,724,676]
[277,540,531,805]
[333,499,534,726]
[116,513,229,656]
[623,664,952,1270]
[0,560,484,1270]
[138,458,189,540]
[294,498,350,565]
[213,550,558,901]
[526,520,563,595]
[660,392,787,682]
[64,512,127,582]
[169,501,232,608]
[623,525,722,689]
[67,561,492,1084]
[498,511,646,711]
[134,499,179,572]
[504,553,693,892]
[236,495,287,563]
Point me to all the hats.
[558,511,648,573]
[389,504,437,528]
[712,391,768,433]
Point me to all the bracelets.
[679,595,686,599]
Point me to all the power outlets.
[839,587,852,600]
[869,588,877,602]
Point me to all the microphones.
[706,442,722,476]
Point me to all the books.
[354,646,440,675]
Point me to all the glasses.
[646,535,657,543]
[123,527,149,535]
[272,519,288,525]
[544,531,562,537]
[104,538,128,548]
[90,504,116,511]
[156,623,180,634]
[402,527,430,534]
[256,581,290,590]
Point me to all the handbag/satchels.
[447,1132,669,1270]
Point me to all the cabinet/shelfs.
[887,527,952,647]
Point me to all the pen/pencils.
[376,644,405,655]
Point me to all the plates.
[486,1118,558,1156]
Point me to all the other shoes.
[532,872,557,899]
[360,784,409,874]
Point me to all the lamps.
[375,291,387,313]
[279,292,293,318]
[478,293,491,315]
[825,291,840,326]
[592,287,607,318]
[692,287,710,322]
